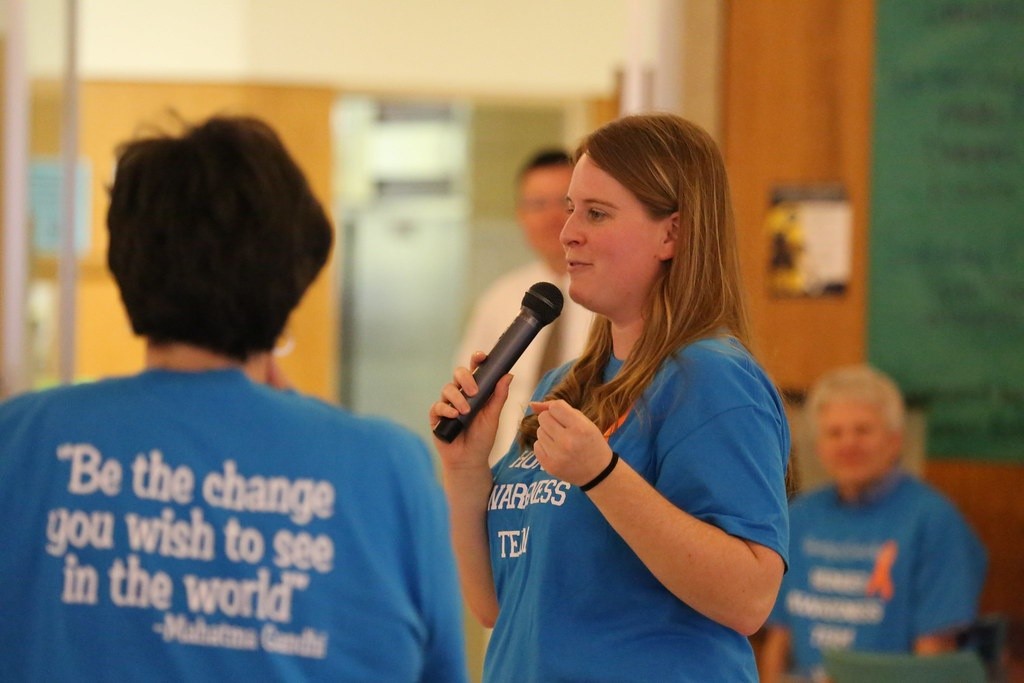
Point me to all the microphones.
[431,282,563,444]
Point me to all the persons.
[763,363,986,683]
[0,115,469,683]
[430,114,792,683]
[451,148,597,466]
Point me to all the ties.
[537,321,562,384]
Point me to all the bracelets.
[580,452,620,493]
